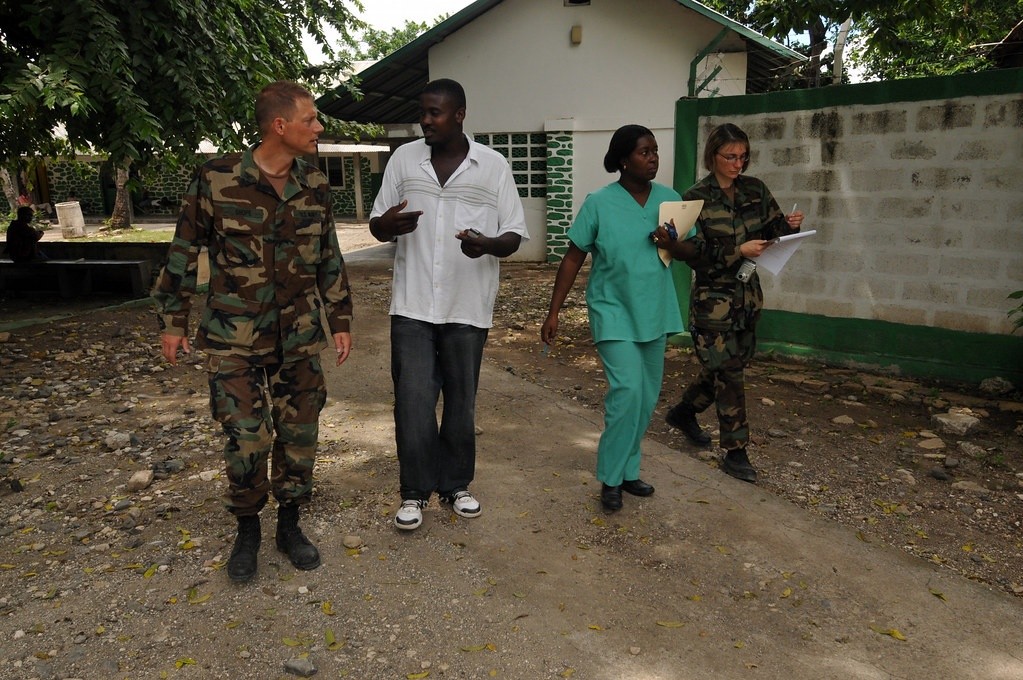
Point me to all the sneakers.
[438,490,482,518]
[394,498,428,530]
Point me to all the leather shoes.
[723,449,757,482]
[624,479,655,497]
[601,482,623,509]
[666,401,712,447]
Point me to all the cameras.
[735,260,757,283]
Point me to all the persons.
[151,83,352,579]
[370,78,528,529]
[7,208,50,267]
[665,123,805,483]
[541,124,701,510]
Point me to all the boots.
[276,503,321,570]
[228,515,261,582]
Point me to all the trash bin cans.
[54,200,86,239]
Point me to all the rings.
[654,237,658,243]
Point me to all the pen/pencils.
[790,202,798,216]
[543,343,548,353]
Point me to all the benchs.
[0,257,152,295]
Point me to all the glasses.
[716,152,749,162]
